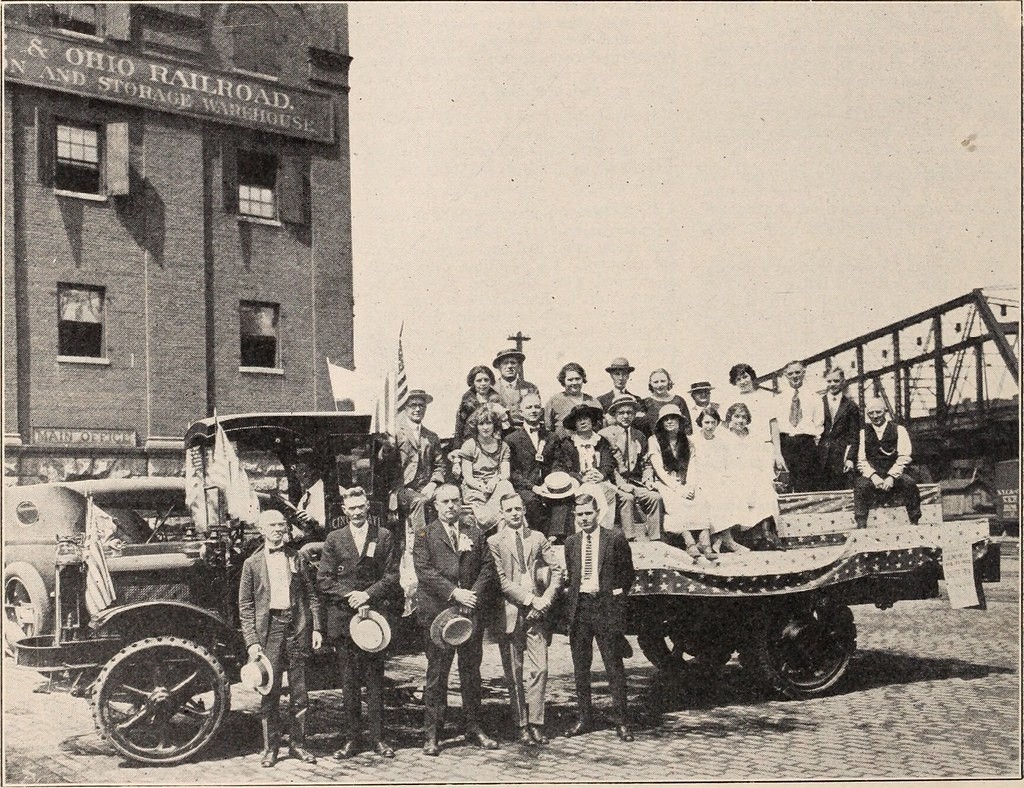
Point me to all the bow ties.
[529,426,540,433]
[268,546,284,554]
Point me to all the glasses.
[613,410,636,417]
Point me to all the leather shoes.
[261,718,634,767]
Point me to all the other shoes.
[910,518,918,526]
[682,530,787,560]
[856,513,868,530]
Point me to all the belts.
[270,607,292,617]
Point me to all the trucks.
[5,408,1003,766]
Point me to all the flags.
[181,422,259,529]
[330,364,374,402]
[85,537,115,616]
[374,340,409,436]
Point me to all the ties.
[450,529,461,552]
[829,397,837,432]
[514,531,526,575]
[413,426,421,448]
[583,534,592,581]
[789,387,802,427]
[623,426,630,478]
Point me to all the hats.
[615,632,634,659]
[396,389,433,414]
[532,471,580,499]
[430,606,479,650]
[562,404,603,429]
[532,559,551,598]
[653,405,690,429]
[687,380,716,395]
[350,611,392,654]
[608,393,641,417]
[492,348,526,369]
[605,358,636,376]
[240,652,275,695]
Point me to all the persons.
[280,457,325,542]
[854,399,922,529]
[239,508,324,766]
[451,346,860,561]
[485,492,563,745]
[413,484,498,756]
[378,389,445,535]
[562,494,636,741]
[317,486,401,759]
